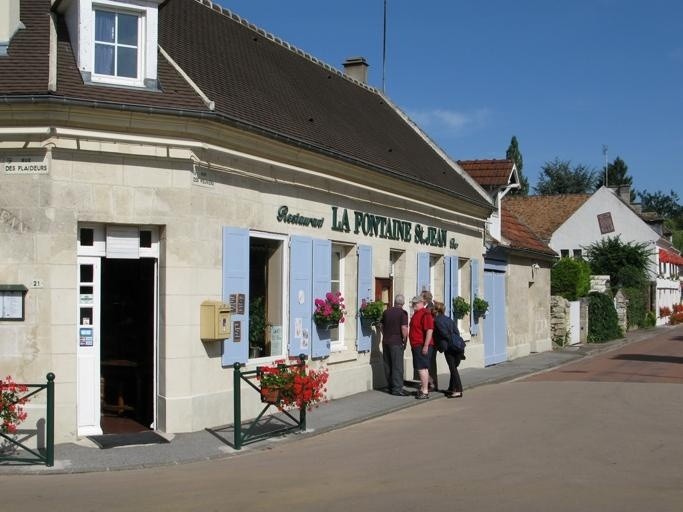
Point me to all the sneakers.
[389,385,434,399]
[445,391,463,398]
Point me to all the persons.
[430,299,465,398]
[420,291,438,392]
[409,295,435,400]
[379,294,409,396]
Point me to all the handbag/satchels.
[449,333,466,355]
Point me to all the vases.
[364,316,378,327]
[263,386,281,402]
[319,318,339,329]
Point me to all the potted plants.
[249,296,270,357]
[452,296,471,319]
[474,297,489,318]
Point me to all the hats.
[409,296,422,303]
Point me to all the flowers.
[362,300,384,322]
[312,291,347,324]
[257,356,331,412]
[0,377,31,434]
